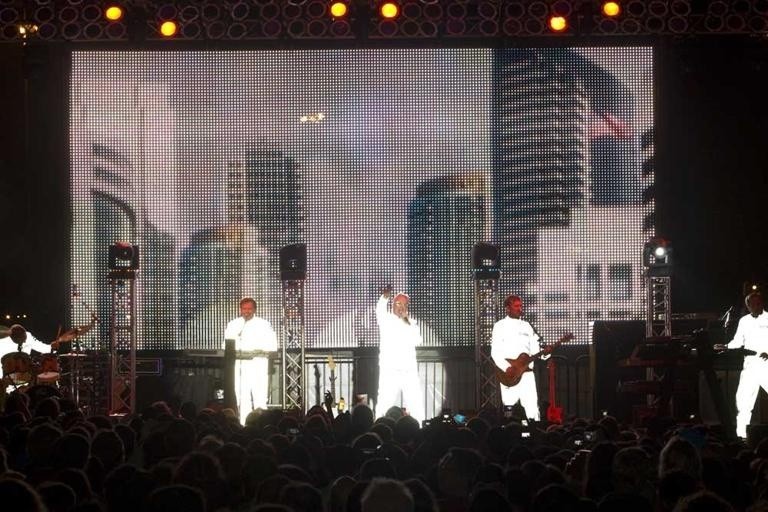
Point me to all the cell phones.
[522,420,528,426]
[521,432,530,437]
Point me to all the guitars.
[495,334,574,386]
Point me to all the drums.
[30,352,63,380]
[21,384,64,416]
[1,352,33,385]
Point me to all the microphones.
[722,305,735,320]
[403,317,410,325]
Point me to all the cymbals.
[57,325,91,343]
[60,352,88,357]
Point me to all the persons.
[722,285,768,439]
[374,285,425,424]
[221,296,278,423]
[489,293,554,421]
[0,323,58,394]
[0,379,767,512]
